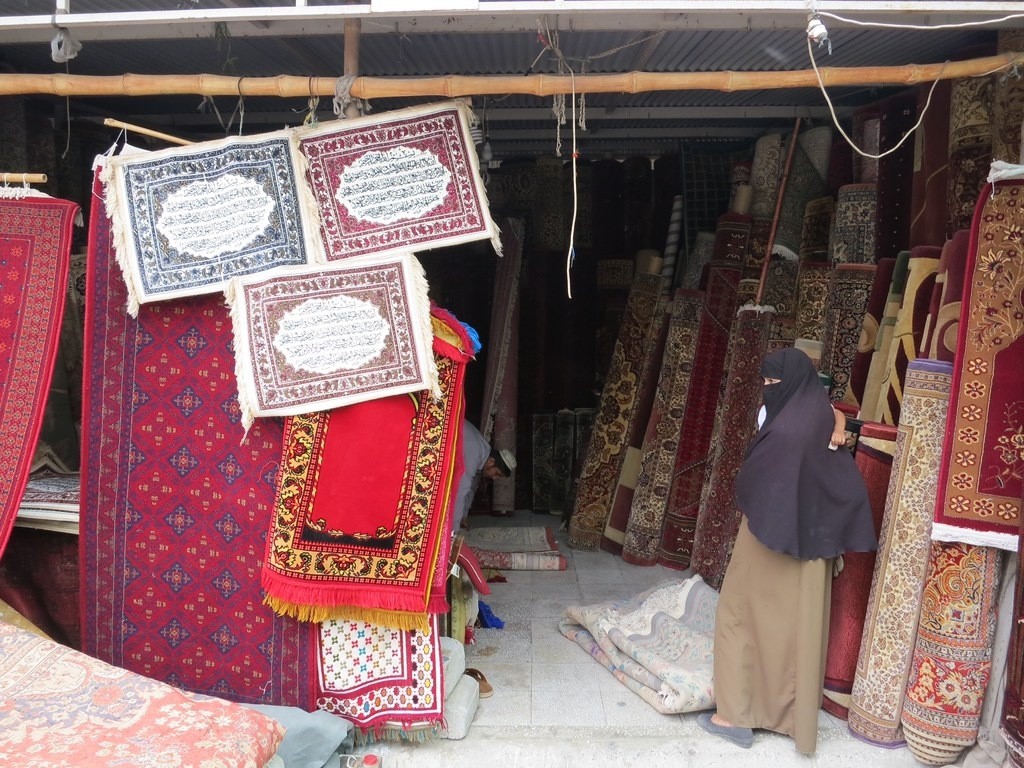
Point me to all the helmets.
[500,448,516,472]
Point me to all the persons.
[452,417,518,544]
[695,345,881,755]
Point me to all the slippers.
[463,668,492,698]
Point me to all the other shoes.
[697,713,755,748]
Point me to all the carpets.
[0,619,286,767]
[0,186,83,560]
[464,527,565,571]
[535,28,1024,768]
[480,217,525,515]
[77,154,311,713]
[222,252,442,446]
[99,121,317,320]
[260,299,481,632]
[315,614,447,745]
[289,103,503,264]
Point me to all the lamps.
[468,104,482,145]
[478,104,490,185]
[481,103,492,161]
